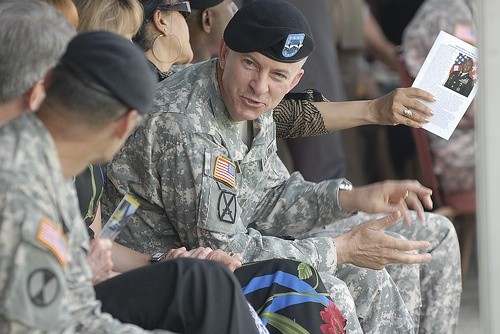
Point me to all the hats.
[224,0,314,63]
[63,30,155,114]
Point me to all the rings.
[402,109,413,118]
[228,252,235,257]
[402,189,408,200]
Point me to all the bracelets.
[339,183,353,190]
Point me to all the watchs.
[149,250,168,263]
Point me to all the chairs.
[397,60,476,275]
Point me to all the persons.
[444,59,474,97]
[0,29,260,334]
[0,0,478,334]
